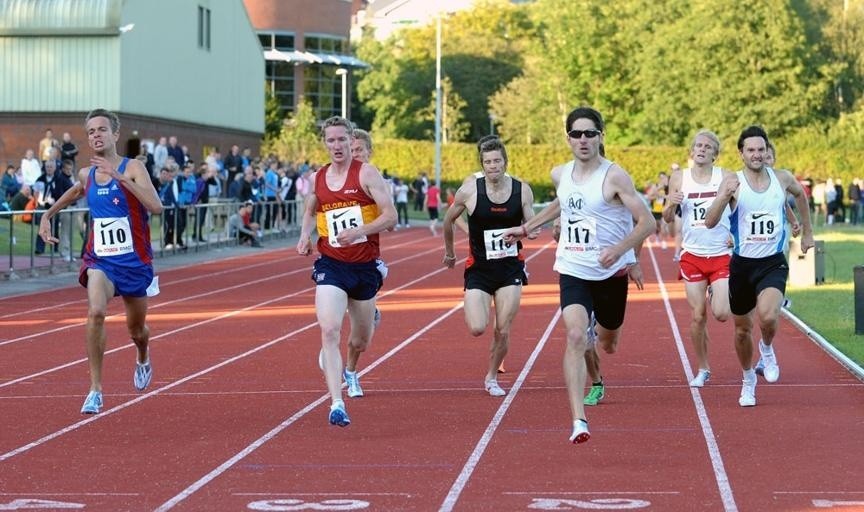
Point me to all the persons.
[136,136,319,251]
[449,134,509,373]
[441,138,542,396]
[295,115,398,428]
[662,131,733,388]
[38,109,164,414]
[317,128,397,371]
[550,142,646,407]
[502,107,657,445]
[705,125,815,408]
[754,142,800,375]
[643,163,864,262]
[0,128,80,263]
[383,169,441,236]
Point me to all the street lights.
[336,68,348,119]
[427,7,441,188]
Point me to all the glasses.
[568,129,600,139]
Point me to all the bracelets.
[521,226,529,239]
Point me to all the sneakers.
[690,369,711,388]
[134,345,153,391]
[758,339,780,383]
[80,391,103,414]
[755,357,765,375]
[328,402,350,427]
[484,379,506,396]
[586,311,599,351]
[739,371,758,406]
[583,383,604,406]
[342,367,364,397]
[568,419,591,445]
